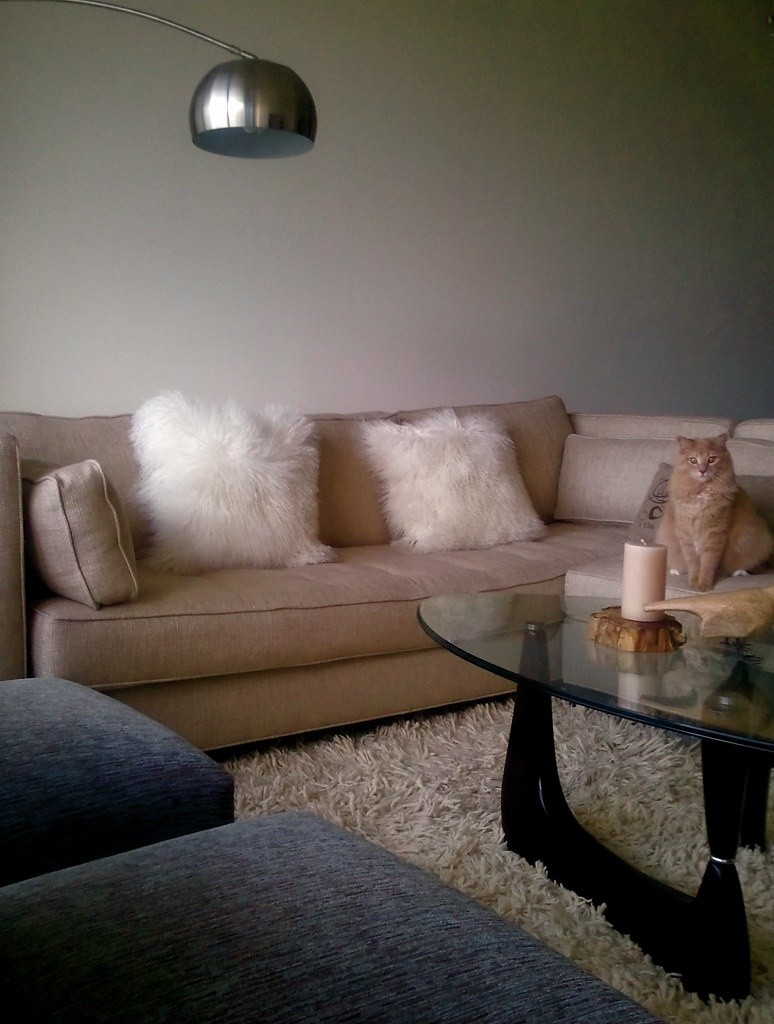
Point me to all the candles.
[621,538,668,622]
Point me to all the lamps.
[46,0,316,159]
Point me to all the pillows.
[725,436,774,522]
[344,405,550,557]
[553,434,678,524]
[128,390,337,575]
[19,459,140,610]
[621,461,774,542]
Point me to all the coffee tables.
[415,591,774,1006]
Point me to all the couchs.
[0,675,236,887]
[0,811,666,1024]
[1,396,774,752]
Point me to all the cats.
[654,432,774,590]
